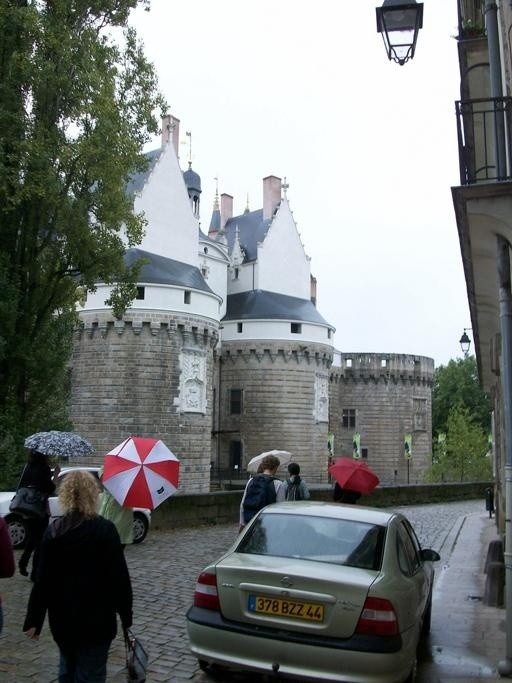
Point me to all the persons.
[21,469,135,683]
[0,516,17,635]
[97,464,134,553]
[283,462,310,501]
[17,448,62,577]
[237,454,288,536]
[332,481,362,504]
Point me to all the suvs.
[0,466,153,551]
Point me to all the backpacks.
[285,478,301,501]
[244,474,280,524]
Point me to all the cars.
[185,498,442,681]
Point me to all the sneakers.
[19,559,29,576]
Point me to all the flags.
[186,130,191,136]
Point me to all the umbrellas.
[325,457,379,495]
[23,430,97,467]
[246,449,291,473]
[102,436,182,511]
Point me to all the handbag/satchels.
[125,639,149,682]
[9,485,52,518]
